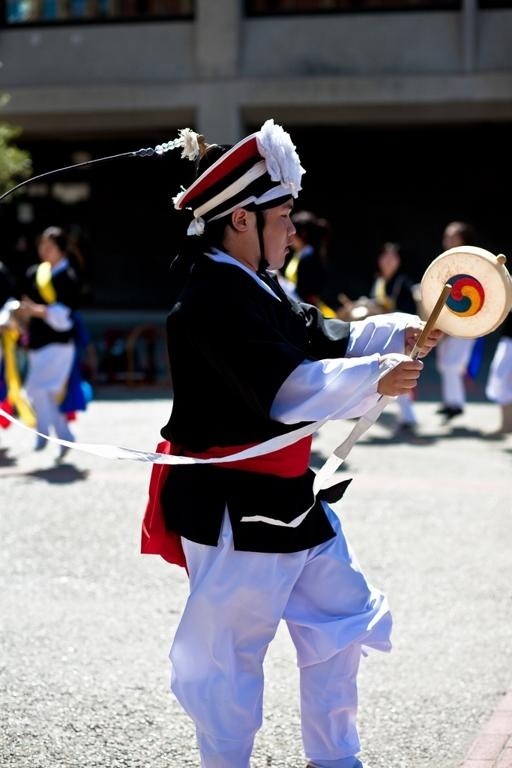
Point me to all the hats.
[174,130,298,226]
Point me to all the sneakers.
[56,447,66,463]
[436,408,462,418]
[34,437,47,450]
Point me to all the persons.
[159,117,441,768]
[14,227,83,460]
